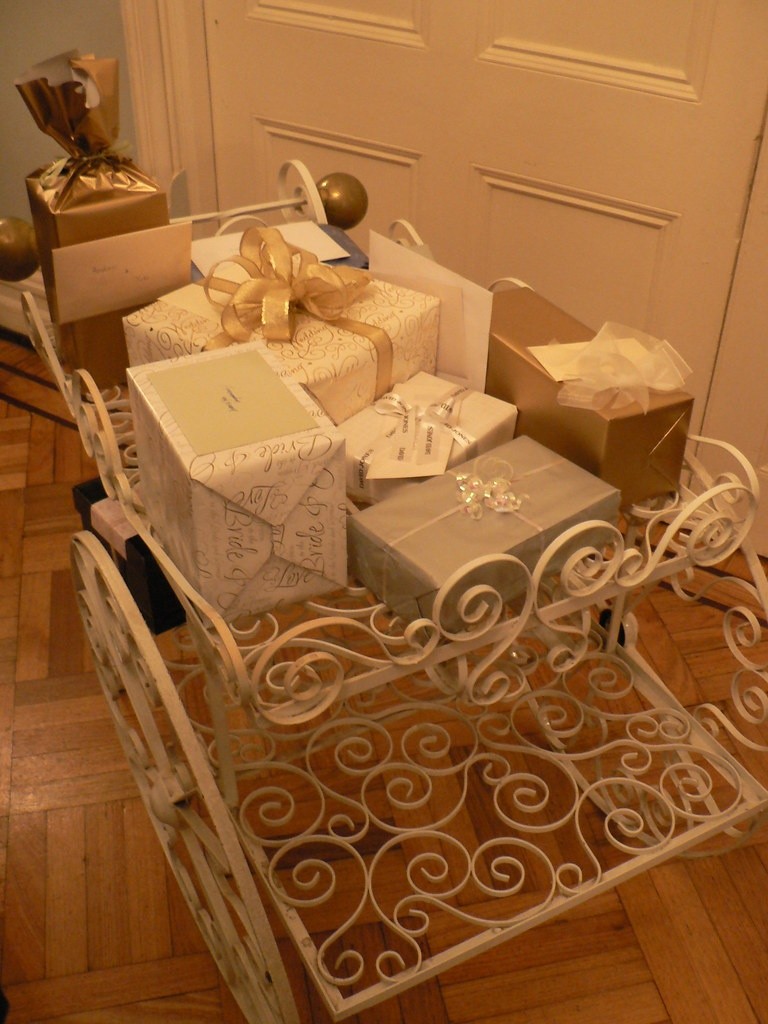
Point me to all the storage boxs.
[21,166,698,644]
